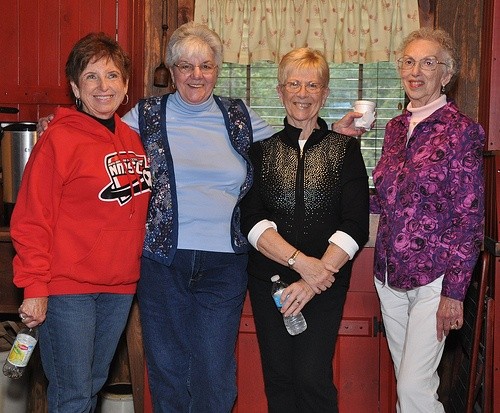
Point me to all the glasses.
[281,80,326,94]
[174,63,218,74]
[397,56,452,72]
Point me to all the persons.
[10,32,152,413]
[35,20,376,413]
[240,47,371,413]
[368,26,486,413]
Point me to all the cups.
[354,100,376,131]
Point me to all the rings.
[294,299,301,305]
[20,314,25,319]
[450,323,458,329]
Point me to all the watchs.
[288,249,301,270]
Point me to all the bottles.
[2,324,39,379]
[270,274,307,335]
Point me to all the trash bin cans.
[99,383,134,413]
